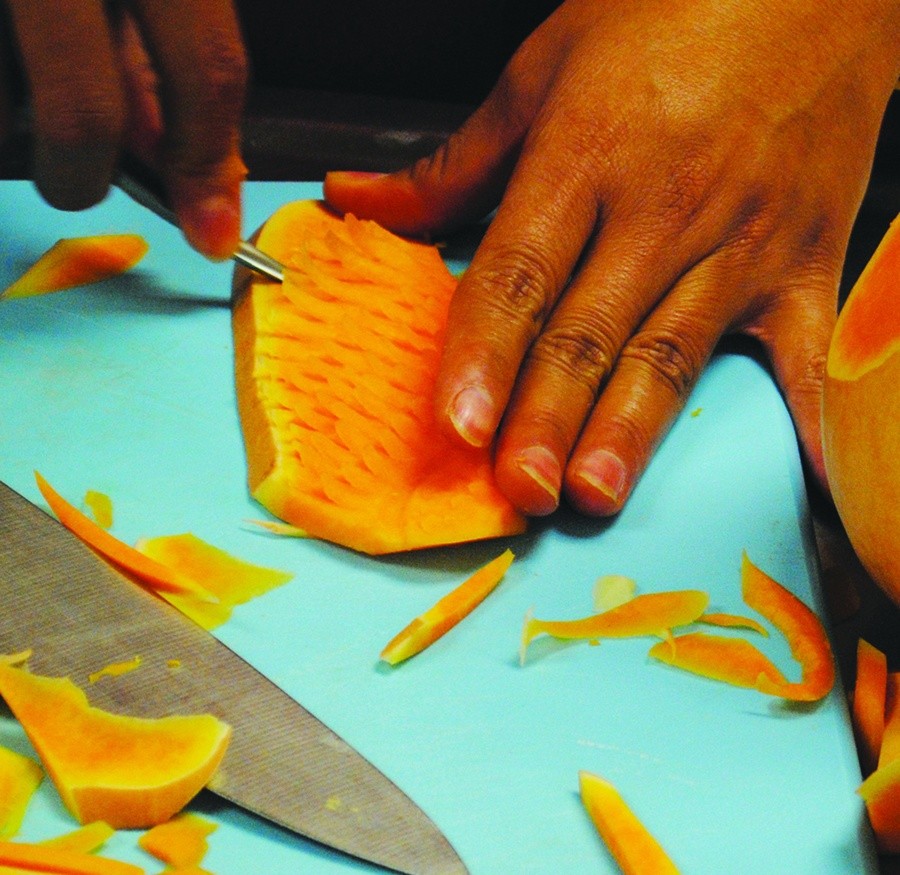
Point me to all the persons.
[0,0,900,517]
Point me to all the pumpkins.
[233,199,528,557]
[0,659,231,832]
[818,208,900,614]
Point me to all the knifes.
[1,481,473,875]
